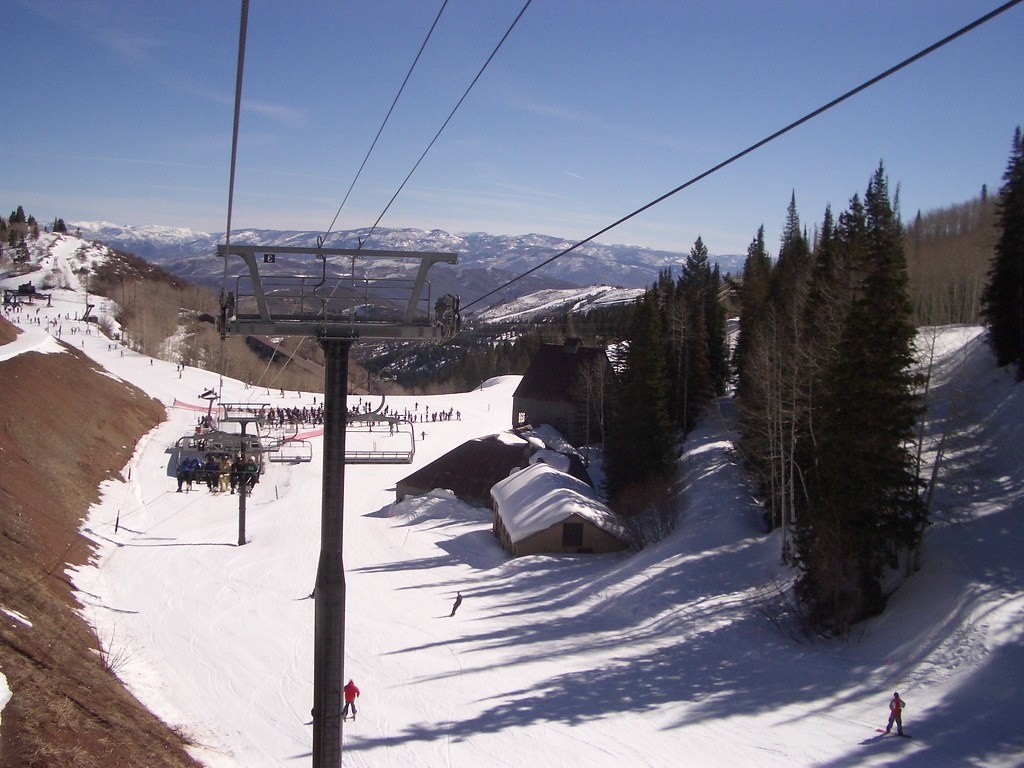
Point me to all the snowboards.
[450,613,454,617]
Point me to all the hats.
[249,456,255,463]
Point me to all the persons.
[344,680,359,720]
[220,378,461,440]
[150,358,153,366]
[107,343,124,357]
[449,591,462,618]
[886,692,905,736]
[313,396,317,405]
[176,455,258,494]
[4,306,92,348]
[176,364,185,378]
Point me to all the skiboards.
[166,485,251,498]
[343,715,356,722]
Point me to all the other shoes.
[353,710,357,714]
[898,733,903,736]
[887,727,890,731]
